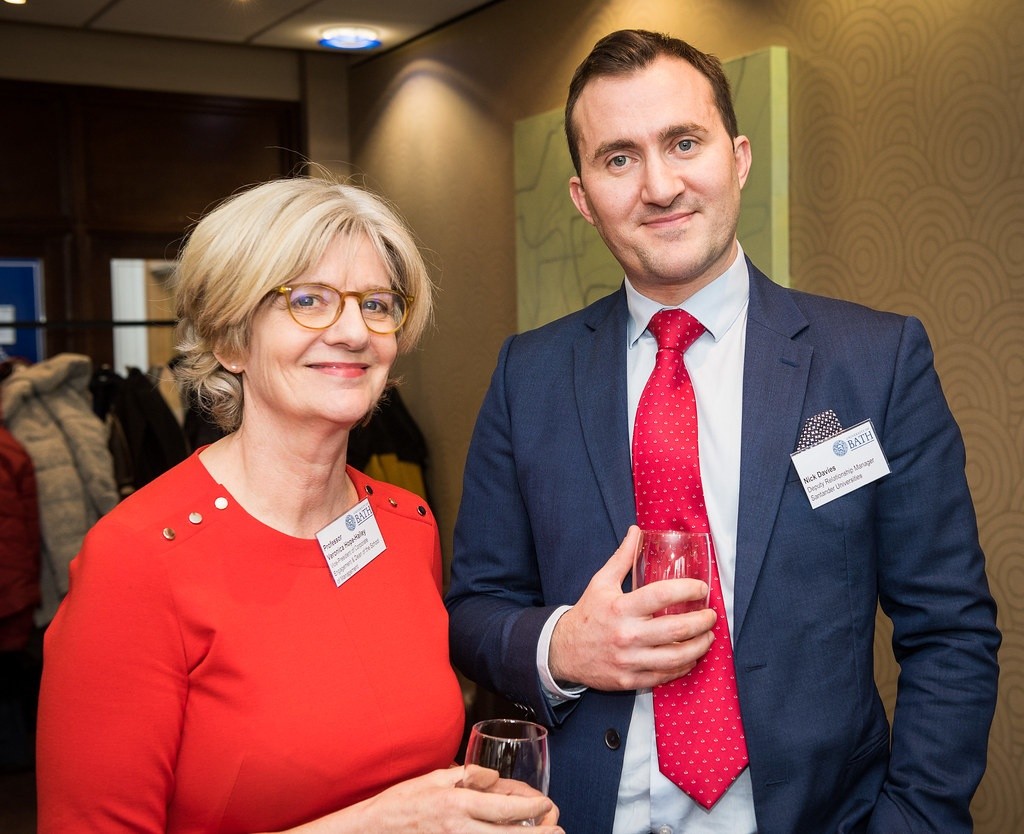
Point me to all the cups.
[464,719,551,826]
[631,531,710,646]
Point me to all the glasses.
[271,282,414,335]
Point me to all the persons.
[441,27,1007,834]
[34,160,569,834]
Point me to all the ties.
[630,308,750,811]
